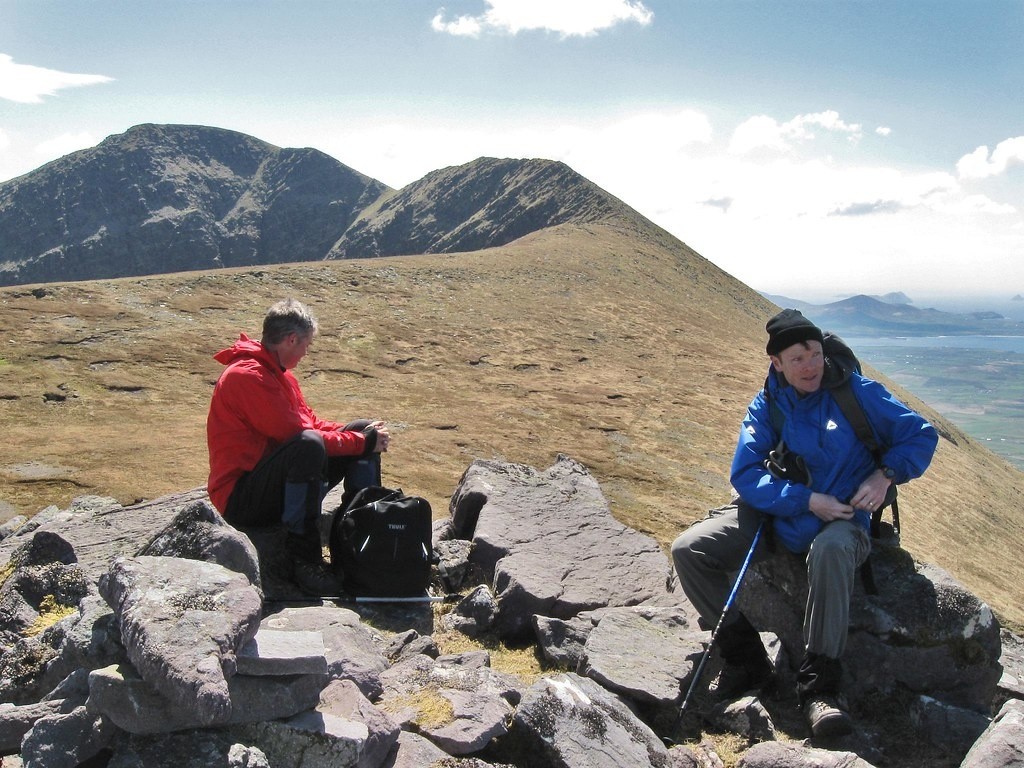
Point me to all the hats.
[765,310,825,355]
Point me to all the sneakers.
[705,657,776,703]
[287,553,344,596]
[801,695,852,737]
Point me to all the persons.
[671,309,939,737]
[207,298,392,596]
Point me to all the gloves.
[763,440,813,489]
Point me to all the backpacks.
[763,331,899,512]
[329,485,434,597]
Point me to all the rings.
[380,426,383,429]
[869,502,873,506]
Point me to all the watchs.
[879,464,898,483]
